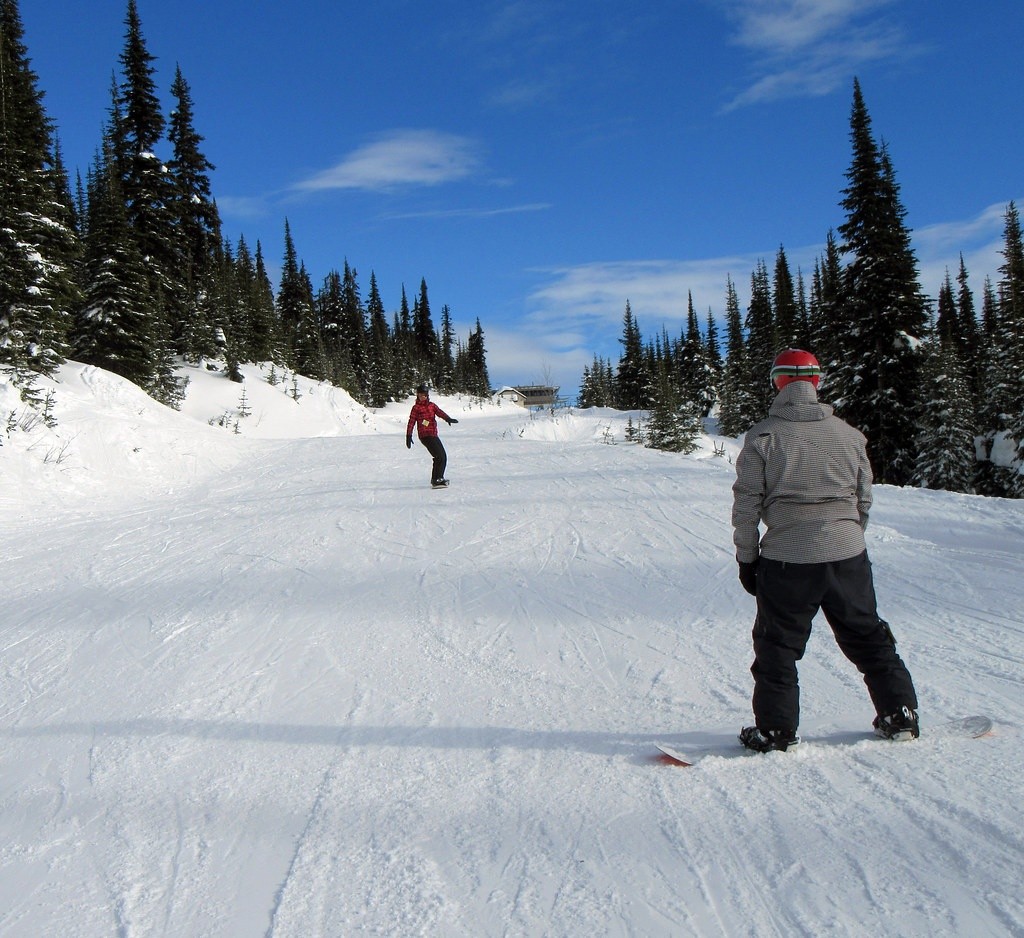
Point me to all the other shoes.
[431,479,448,486]
[739,727,801,751]
[872,706,919,742]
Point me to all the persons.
[406,385,458,486]
[732,349,919,751]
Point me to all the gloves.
[406,436,414,449]
[735,553,758,596]
[446,417,458,426]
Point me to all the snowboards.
[652,714,993,766]
[430,479,450,489]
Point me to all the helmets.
[417,385,429,395]
[770,349,820,389]
